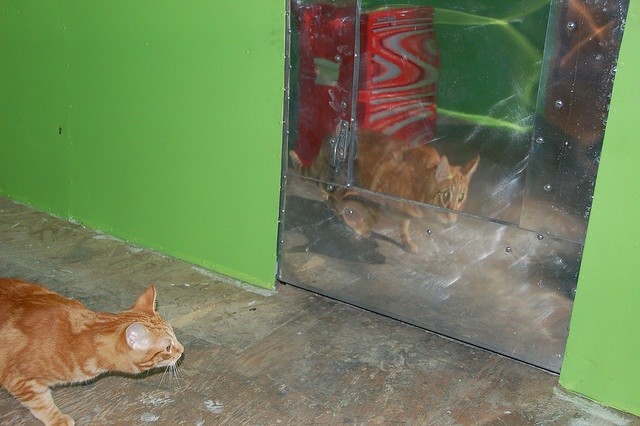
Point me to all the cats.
[290,128,481,254]
[0,277,185,425]
